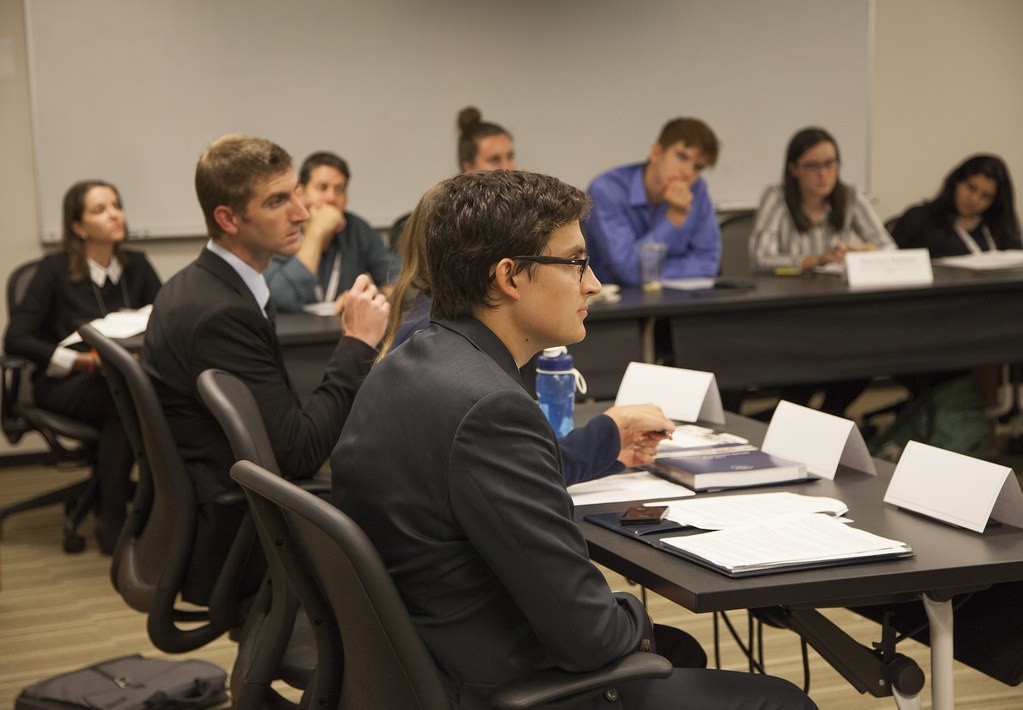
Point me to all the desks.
[109,238,1022,710]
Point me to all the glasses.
[800,158,835,173]
[490,256,590,282]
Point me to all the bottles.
[535,344,578,441]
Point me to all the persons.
[141,137,388,610]
[5,183,166,559]
[263,108,1023,489]
[329,166,822,710]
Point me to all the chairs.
[197,365,326,707]
[227,459,676,710]
[1,261,142,554]
[74,328,244,652]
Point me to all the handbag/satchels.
[15,653,229,710]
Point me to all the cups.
[640,242,664,292]
